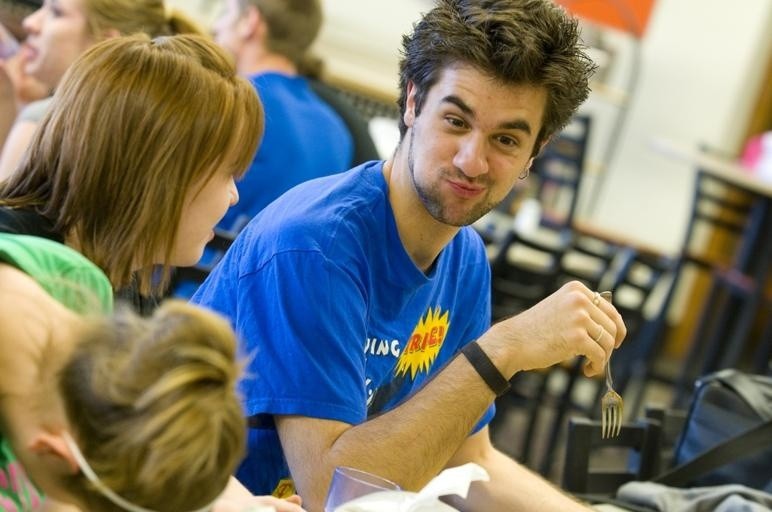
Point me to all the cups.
[323,467,403,512]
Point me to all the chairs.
[488,112,771,495]
[161,229,243,300]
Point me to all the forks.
[599,290,624,439]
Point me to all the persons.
[0,227,250,512]
[180,0,632,512]
[0,28,312,512]
[1,0,399,297]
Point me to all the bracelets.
[456,338,512,401]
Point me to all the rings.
[591,290,603,307]
[595,325,605,345]
